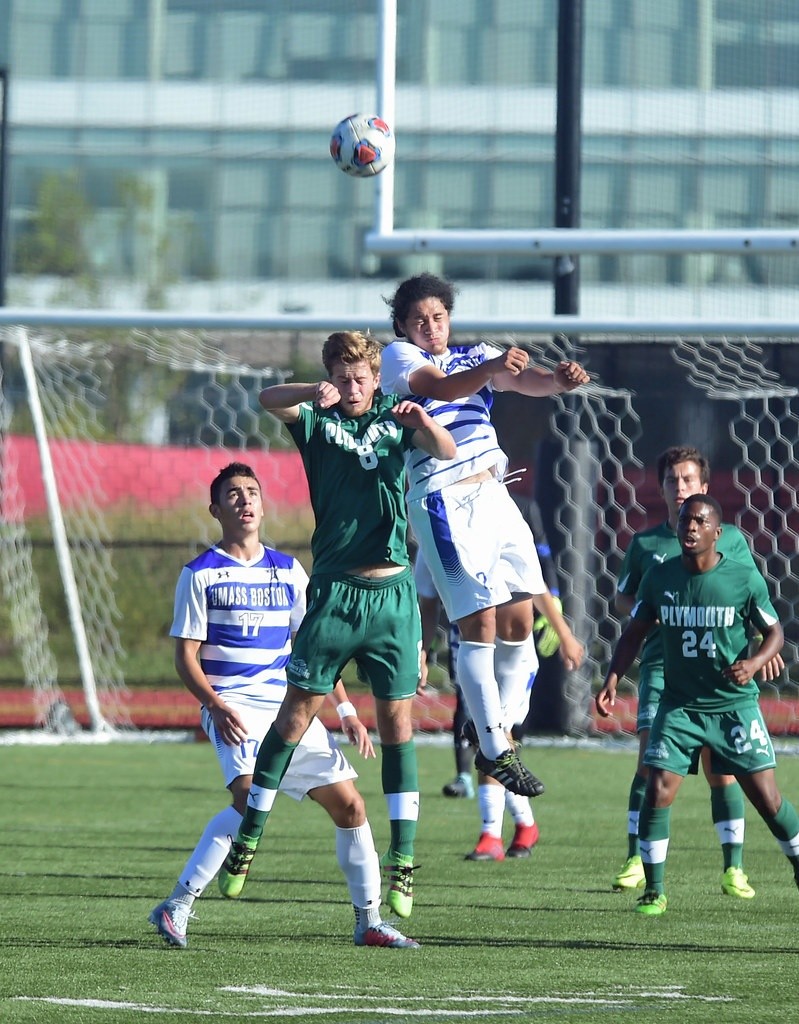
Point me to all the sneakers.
[459,717,481,751]
[443,776,475,797]
[611,855,645,892]
[474,742,545,797]
[147,900,200,949]
[720,866,756,900]
[633,888,668,914]
[353,920,420,949]
[464,833,506,861]
[218,828,261,899]
[379,844,422,918]
[505,822,540,858]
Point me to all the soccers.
[329,110,396,178]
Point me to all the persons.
[591,493,797,915]
[609,445,787,897]
[223,332,455,917]
[413,450,585,862]
[377,275,594,797]
[143,459,422,950]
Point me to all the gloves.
[532,595,564,658]
